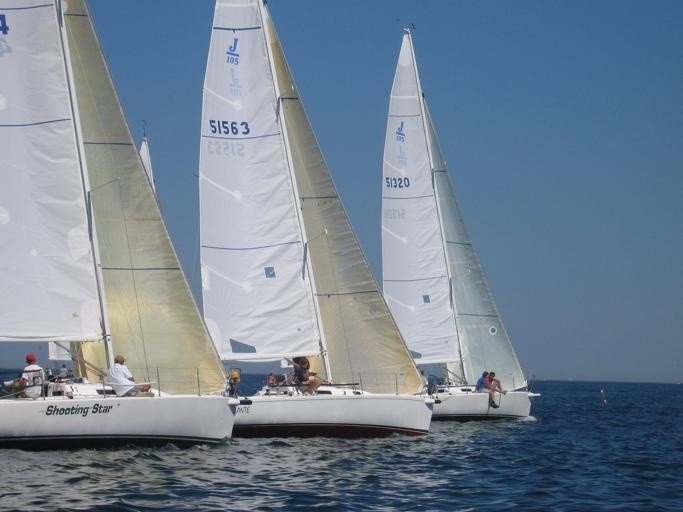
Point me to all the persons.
[58,363,67,378]
[419,369,428,388]
[11,352,44,398]
[227,368,240,390]
[297,359,323,397]
[486,371,507,395]
[267,372,275,385]
[474,370,499,408]
[106,354,154,397]
[287,357,309,397]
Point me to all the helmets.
[113,355,126,363]
[26,354,37,363]
[229,371,240,381]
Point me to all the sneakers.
[490,401,499,409]
